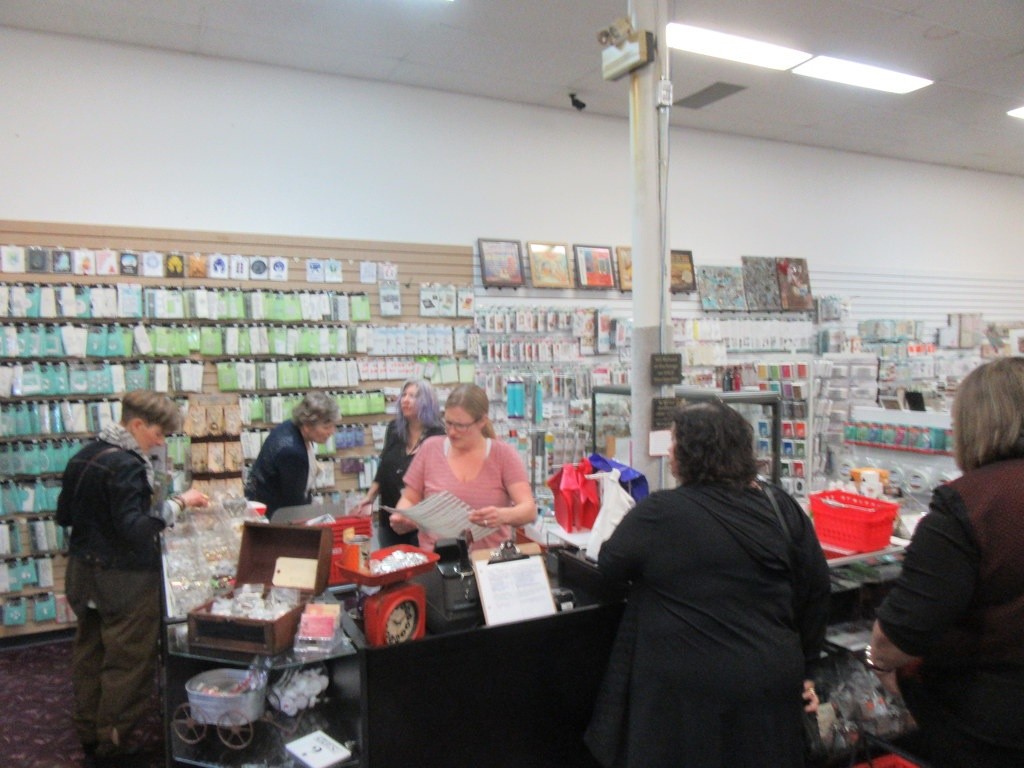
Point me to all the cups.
[342,535,371,574]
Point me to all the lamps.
[597,17,654,81]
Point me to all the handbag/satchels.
[587,452,649,504]
[800,700,851,765]
[584,468,636,563]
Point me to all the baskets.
[808,490,900,552]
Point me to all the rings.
[807,688,816,695]
[484,520,488,525]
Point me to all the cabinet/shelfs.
[159,592,369,768]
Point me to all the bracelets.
[170,495,186,512]
[865,644,894,673]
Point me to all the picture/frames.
[477,238,527,287]
[528,242,575,290]
[669,250,697,291]
[574,244,617,288]
[615,247,632,291]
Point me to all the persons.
[389,381,538,555]
[869,356,1024,768]
[355,376,446,549]
[54,388,209,767]
[242,391,343,522]
[583,397,833,768]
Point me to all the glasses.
[447,416,482,433]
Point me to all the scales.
[335,544,442,646]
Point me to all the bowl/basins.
[185,670,267,726]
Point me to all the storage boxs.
[185,668,267,726]
[187,520,332,655]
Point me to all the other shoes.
[83,741,155,766]
